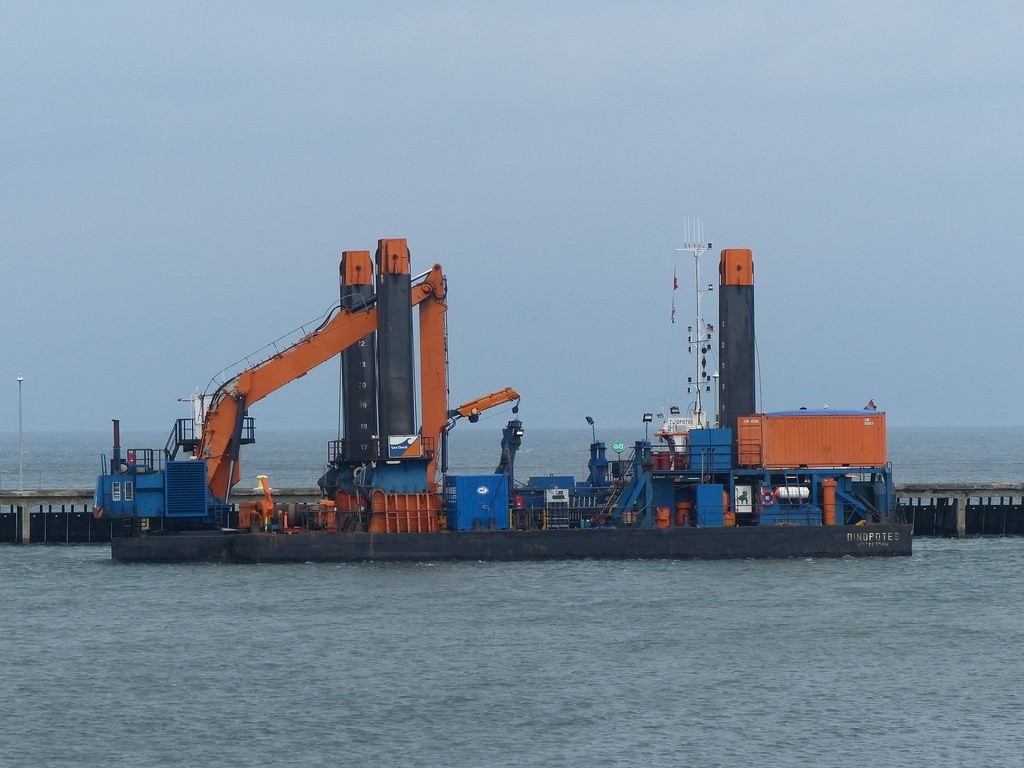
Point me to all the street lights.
[16,371,22,493]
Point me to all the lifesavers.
[758,489,776,507]
[353,467,364,478]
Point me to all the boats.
[93,216,917,563]
